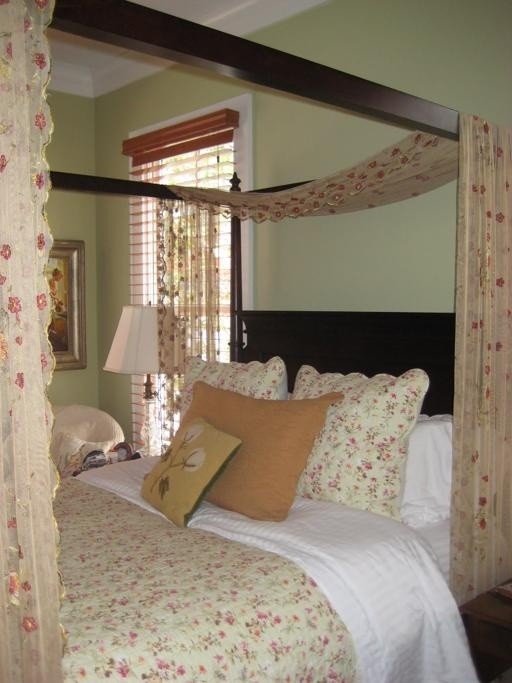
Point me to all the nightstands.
[455,575,511,683]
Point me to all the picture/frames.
[46,238,88,371]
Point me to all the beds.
[0,0,512,683]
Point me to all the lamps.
[102,305,161,399]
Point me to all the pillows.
[289,363,431,525]
[142,410,244,529]
[178,356,290,426]
[178,379,346,524]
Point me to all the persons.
[48,267,65,334]
